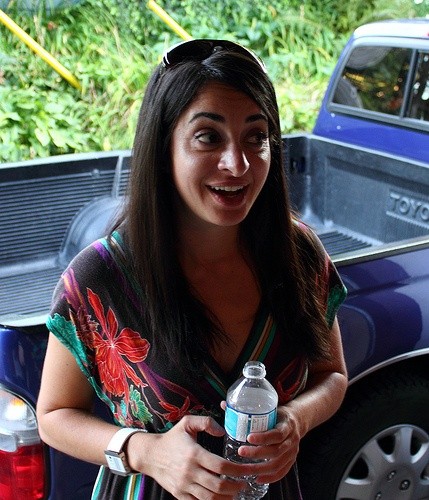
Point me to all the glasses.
[155,39,263,82]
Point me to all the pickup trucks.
[0,19,429,500]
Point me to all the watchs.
[106,426,147,477]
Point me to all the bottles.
[221,361,279,500]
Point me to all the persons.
[34,38,349,500]
